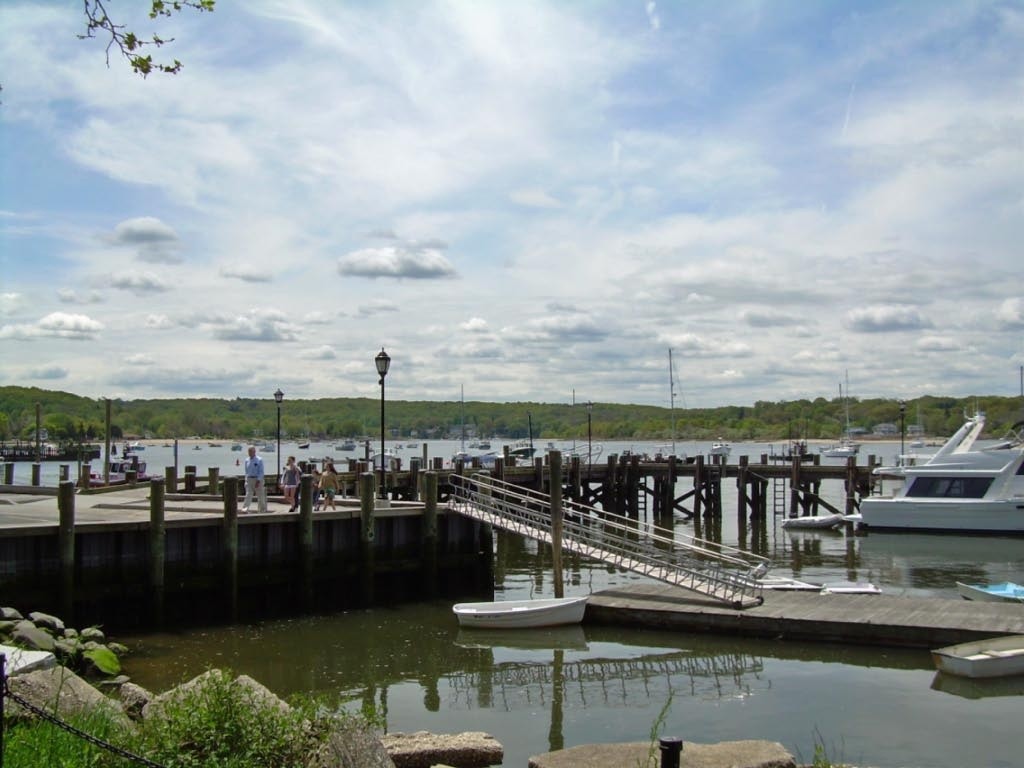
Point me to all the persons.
[280,456,339,512]
[239,446,264,512]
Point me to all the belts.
[248,476,258,478]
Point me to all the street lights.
[898,398,906,467]
[273,388,284,492]
[375,346,392,499]
[585,399,595,478]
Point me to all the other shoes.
[314,506,319,511]
[240,508,248,514]
[289,508,295,512]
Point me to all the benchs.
[979,649,1001,656]
[459,609,477,612]
[512,607,528,610]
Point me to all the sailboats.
[912,402,923,447]
[639,346,695,463]
[451,384,525,468]
[819,368,861,458]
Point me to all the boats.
[509,438,537,459]
[857,417,1024,537]
[74,453,163,487]
[956,580,1024,602]
[768,416,813,462]
[711,437,732,456]
[371,448,399,469]
[299,443,310,448]
[452,597,589,629]
[336,439,374,452]
[468,438,491,450]
[931,634,1024,679]
[712,570,882,597]
[453,625,586,651]
[232,438,275,452]
[394,442,419,449]
